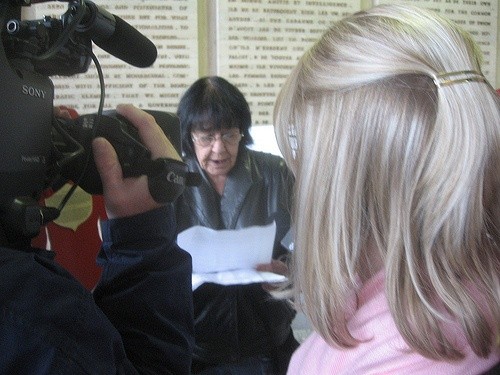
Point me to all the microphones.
[70,1,157,68]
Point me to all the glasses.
[192,132,244,147]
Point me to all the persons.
[0,100,200,375]
[166,73,307,374]
[270,3,500,375]
